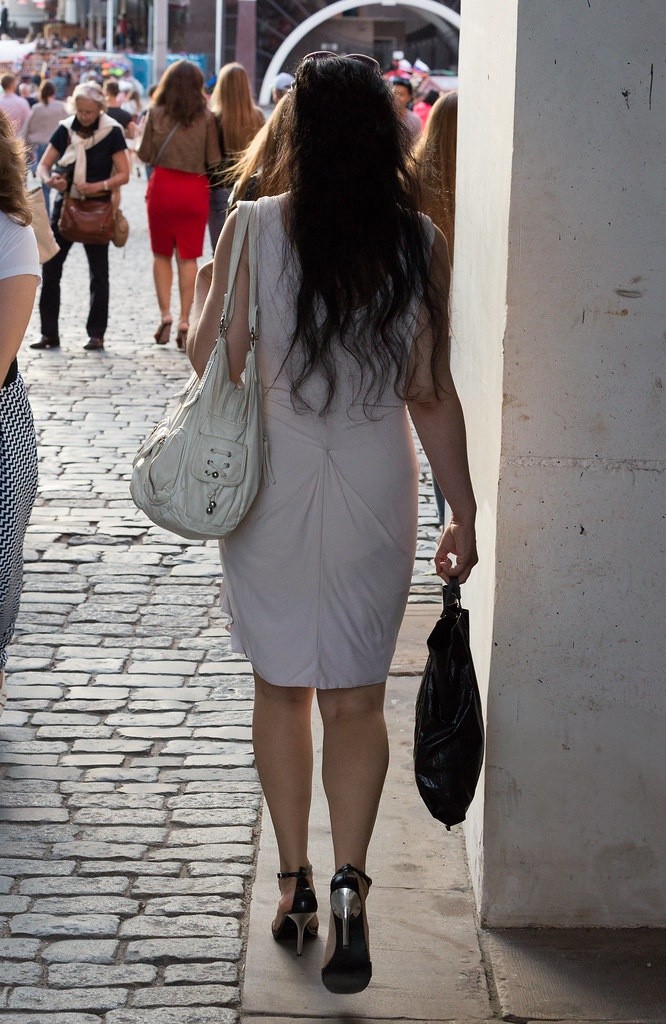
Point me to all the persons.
[186,52,478,995]
[0,12,458,351]
[0,110,38,682]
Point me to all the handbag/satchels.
[145,163,154,180]
[128,338,262,541]
[414,574,483,830]
[24,186,61,264]
[56,196,114,243]
[112,207,129,247]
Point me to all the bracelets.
[104,180,108,190]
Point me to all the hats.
[272,72,297,90]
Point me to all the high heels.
[155,316,171,345]
[273,867,321,954]
[176,323,188,349]
[321,863,373,993]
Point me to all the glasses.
[304,50,381,78]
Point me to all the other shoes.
[85,339,103,350]
[28,336,60,349]
